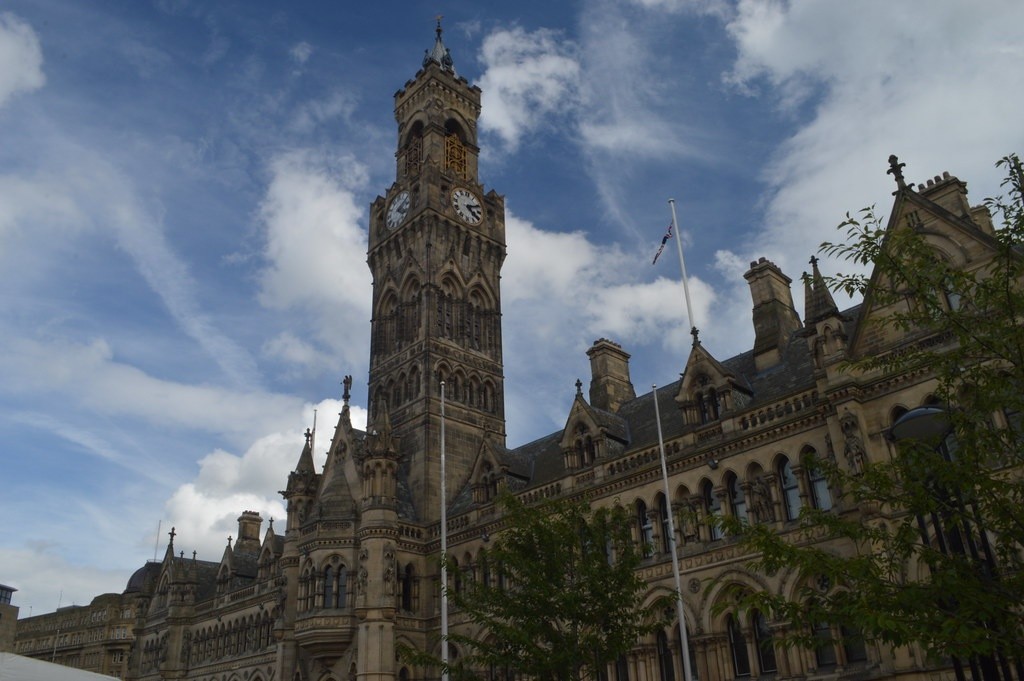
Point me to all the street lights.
[887,404,1003,681]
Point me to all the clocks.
[385,190,411,229]
[451,188,483,225]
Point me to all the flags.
[653,220,673,265]
